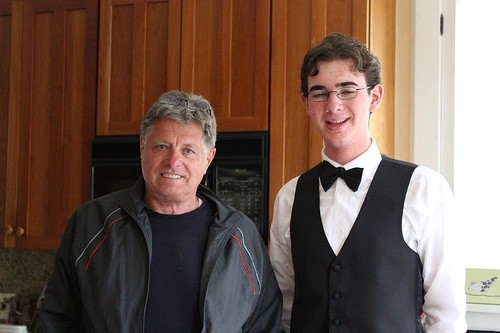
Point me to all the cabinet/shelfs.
[0,0,397,250]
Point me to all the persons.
[269,32,467,333]
[28,91,285,333]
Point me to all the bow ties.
[320,160,364,192]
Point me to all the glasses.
[302,86,372,102]
